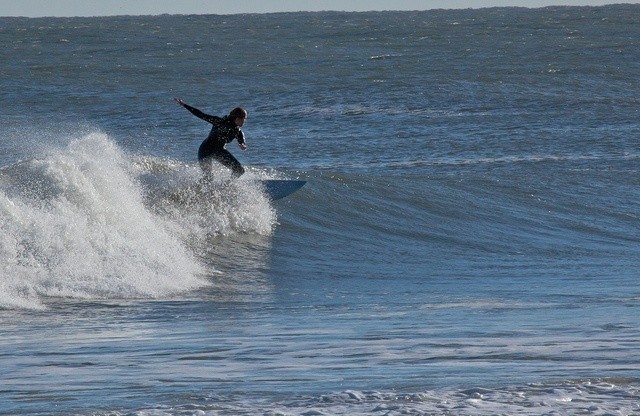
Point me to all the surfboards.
[259,180,307,202]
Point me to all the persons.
[174,98,247,184]
